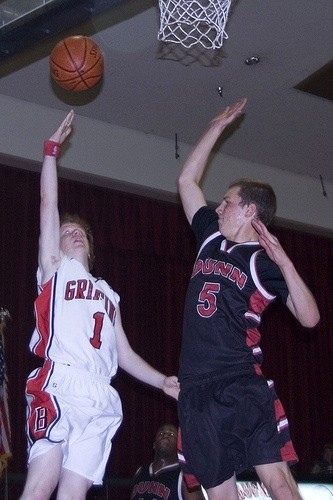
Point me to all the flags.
[0,323,14,475]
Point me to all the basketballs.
[49,35,105,93]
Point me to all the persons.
[128,423,206,500]
[175,97,320,500]
[21,110,179,500]
[311,443,333,473]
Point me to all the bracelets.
[44,139,61,158]
